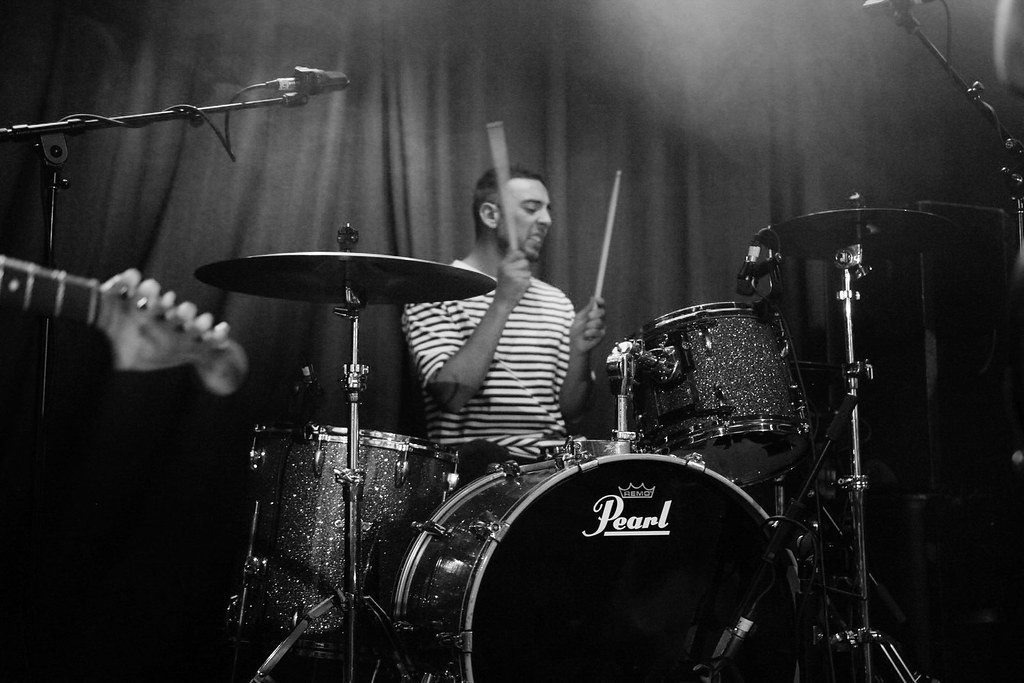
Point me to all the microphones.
[267,69,349,93]
[735,234,761,297]
[862,0,936,15]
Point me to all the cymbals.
[191,249,498,308]
[753,205,958,268]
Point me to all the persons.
[0,269,248,683]
[400,162,606,464]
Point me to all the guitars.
[0,250,249,399]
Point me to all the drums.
[629,298,820,491]
[386,429,824,683]
[221,407,460,683]
